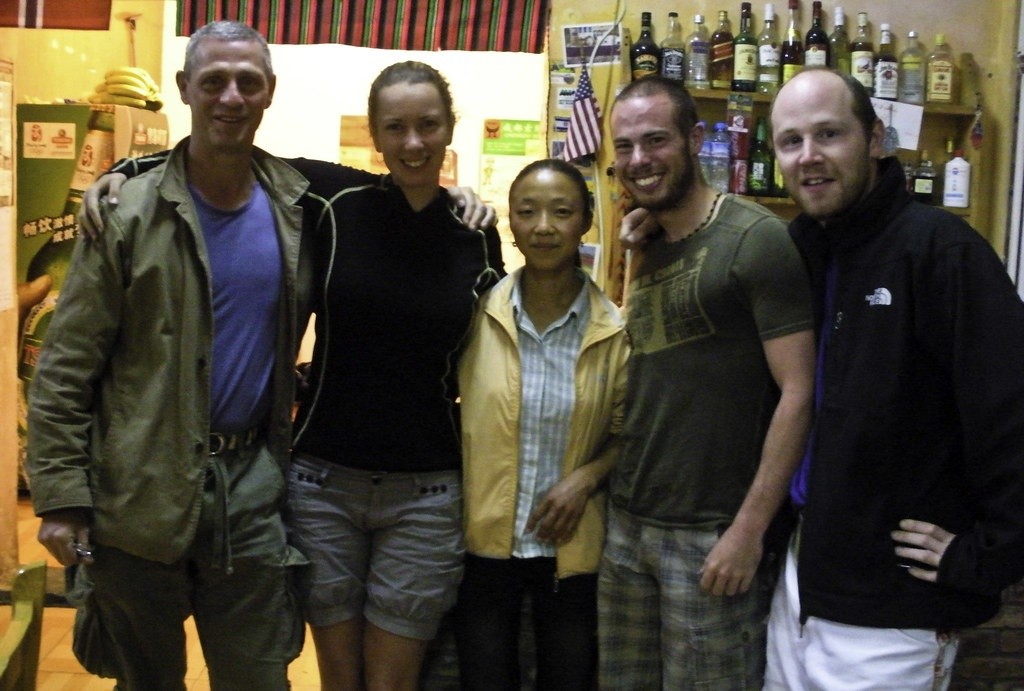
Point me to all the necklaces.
[663,192,722,240]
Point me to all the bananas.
[88,68,163,110]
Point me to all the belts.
[207,426,268,456]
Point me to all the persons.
[24,21,1024,691]
[16,273,51,356]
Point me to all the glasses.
[74,543,96,558]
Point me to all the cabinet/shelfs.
[605,88,993,307]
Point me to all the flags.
[563,57,603,163]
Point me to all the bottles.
[712,123,730,194]
[850,13,874,89]
[872,23,899,100]
[750,117,772,195]
[898,30,928,106]
[711,11,734,89]
[828,4,852,74]
[662,12,687,80]
[913,138,971,208]
[686,14,710,89]
[732,3,758,92]
[695,121,712,185]
[631,12,660,82]
[803,1,829,68]
[774,159,790,196]
[927,34,958,102]
[757,3,781,97]
[17,111,114,488]
[783,0,805,84]
